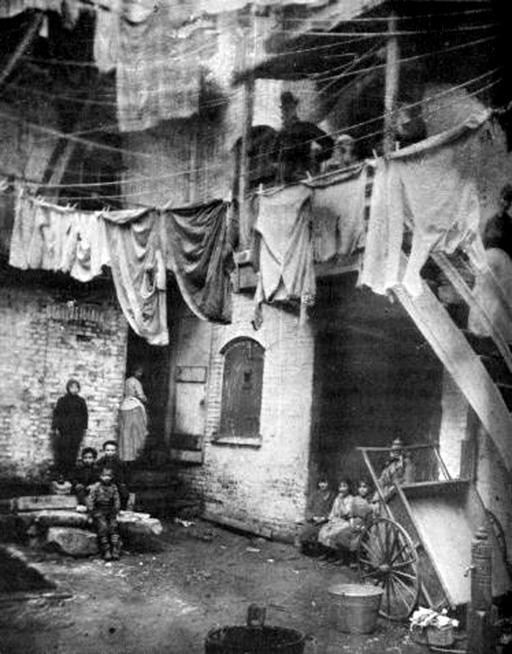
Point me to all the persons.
[74,446,97,496]
[99,444,139,509]
[319,475,353,563]
[336,476,371,569]
[83,466,123,561]
[365,437,417,570]
[265,92,337,184]
[116,363,149,464]
[301,473,335,557]
[51,378,86,481]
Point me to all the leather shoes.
[57,472,65,484]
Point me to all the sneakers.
[76,504,87,513]
[104,550,120,560]
[318,554,358,570]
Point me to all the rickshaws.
[356,443,512,623]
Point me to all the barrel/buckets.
[329,584,383,632]
[204,619,305,654]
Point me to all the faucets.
[463,564,475,577]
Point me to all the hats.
[279,92,300,109]
